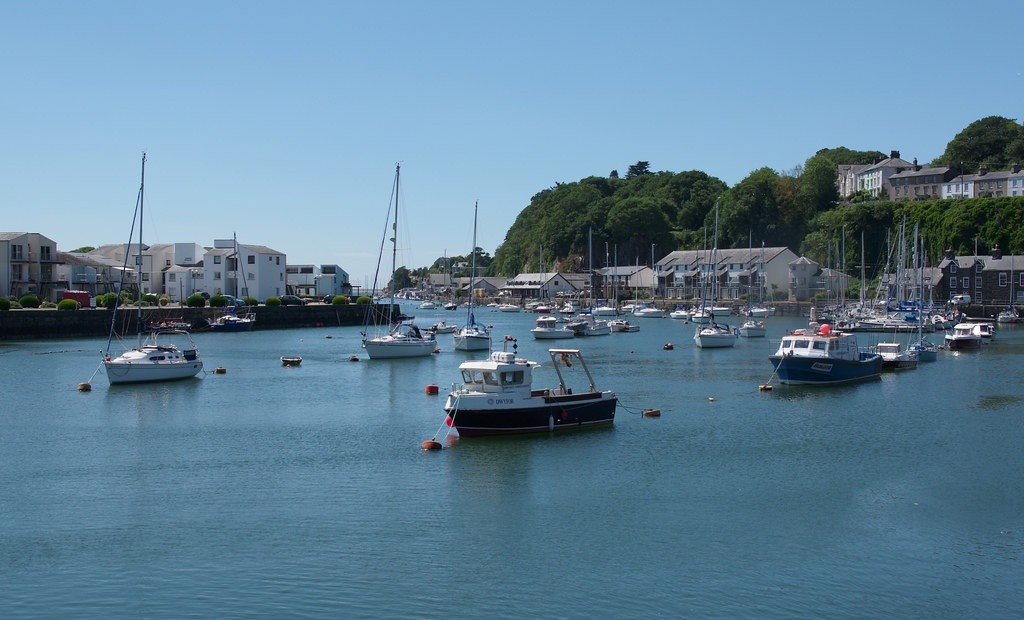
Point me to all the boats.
[143,317,191,333]
[420,321,458,333]
[443,335,618,436]
[419,302,435,310]
[280,355,303,364]
[97,152,206,384]
[767,321,885,386]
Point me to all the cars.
[279,294,308,306]
[220,294,246,307]
[324,294,336,304]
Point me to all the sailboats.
[453,200,493,351]
[487,225,667,339]
[813,218,1019,374]
[444,264,458,310]
[670,201,775,348]
[358,161,439,358]
[206,232,257,332]
